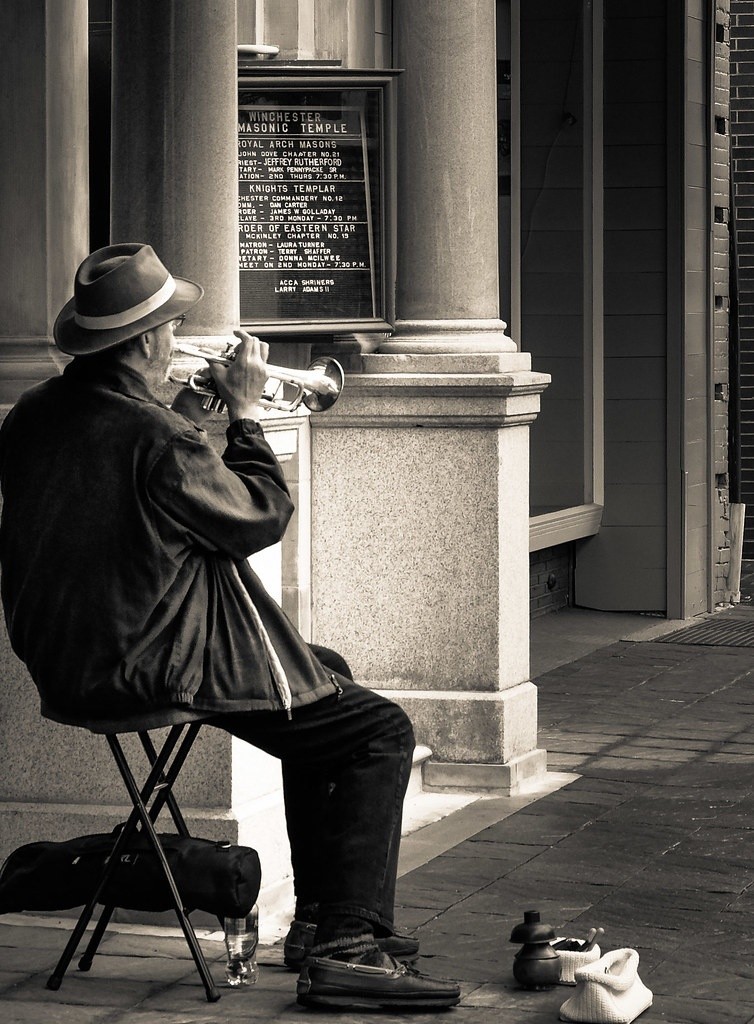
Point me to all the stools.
[36,703,237,1003]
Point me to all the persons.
[0,243,463,1013]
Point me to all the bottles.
[223,902,260,986]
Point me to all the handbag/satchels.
[3,825,261,918]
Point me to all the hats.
[54,241,205,355]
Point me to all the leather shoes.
[285,920,424,969]
[296,948,461,1010]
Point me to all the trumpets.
[169,340,345,417]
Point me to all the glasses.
[172,314,185,333]
